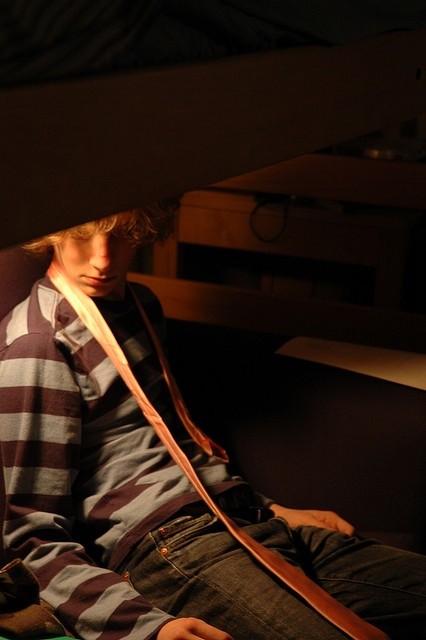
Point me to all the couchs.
[0,243,425,550]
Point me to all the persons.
[0,205,426,640]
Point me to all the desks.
[150,154,426,313]
